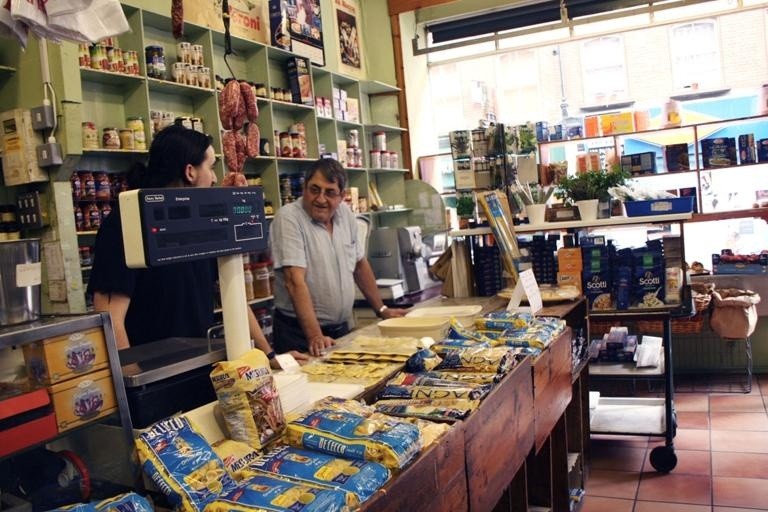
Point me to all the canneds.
[94,169,110,201]
[82,122,99,148]
[121,174,129,195]
[126,115,148,151]
[116,127,134,151]
[69,171,81,199]
[109,172,120,199]
[185,65,199,86]
[74,200,83,231]
[176,42,191,64]
[79,36,140,77]
[99,203,113,219]
[103,126,120,151]
[145,45,166,79]
[80,169,97,201]
[198,67,210,89]
[85,202,101,231]
[170,62,186,85]
[191,44,203,67]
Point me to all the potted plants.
[553,168,638,222]
[455,196,475,230]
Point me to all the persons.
[86,125,313,369]
[268,159,411,354]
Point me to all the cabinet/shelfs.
[404,293,591,441]
[307,351,538,511]
[2,1,416,347]
[338,318,592,512]
[447,122,538,246]
[541,113,768,375]
[0,374,464,512]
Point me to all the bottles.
[381,150,390,169]
[290,130,303,158]
[354,148,362,167]
[77,247,82,267]
[260,138,270,155]
[176,116,191,131]
[265,261,275,293]
[82,246,90,266]
[274,129,280,157]
[252,263,271,298]
[346,147,354,166]
[345,128,359,147]
[247,81,256,96]
[289,122,306,158]
[238,79,245,82]
[283,89,291,102]
[390,150,398,168]
[279,173,290,197]
[372,131,386,149]
[216,74,224,90]
[224,78,235,85]
[279,132,292,157]
[291,171,305,197]
[256,83,266,97]
[274,88,283,100]
[188,117,203,133]
[244,264,254,300]
[369,150,381,168]
[271,86,274,99]
[161,112,174,130]
[150,109,161,139]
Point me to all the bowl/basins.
[378,315,449,340]
[403,303,483,327]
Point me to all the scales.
[117,186,271,430]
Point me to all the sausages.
[233,174,247,186]
[219,86,232,129]
[247,122,260,158]
[234,132,247,173]
[239,82,259,120]
[221,173,234,188]
[226,80,239,115]
[223,130,237,173]
[234,90,246,129]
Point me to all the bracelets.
[376,305,388,315]
[266,351,275,360]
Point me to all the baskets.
[588,320,619,339]
[635,281,710,335]
